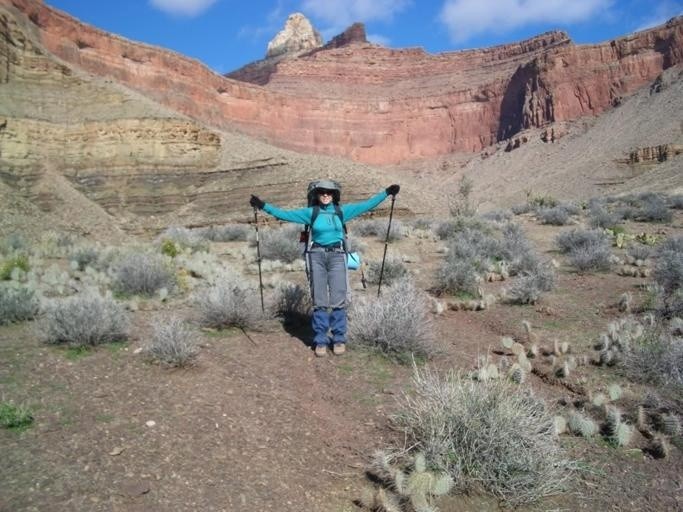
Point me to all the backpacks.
[303,180,348,240]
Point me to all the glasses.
[317,189,335,196]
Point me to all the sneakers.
[314,341,328,357]
[333,342,347,356]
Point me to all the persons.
[248,176,402,357]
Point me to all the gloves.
[249,193,267,211]
[385,184,400,196]
